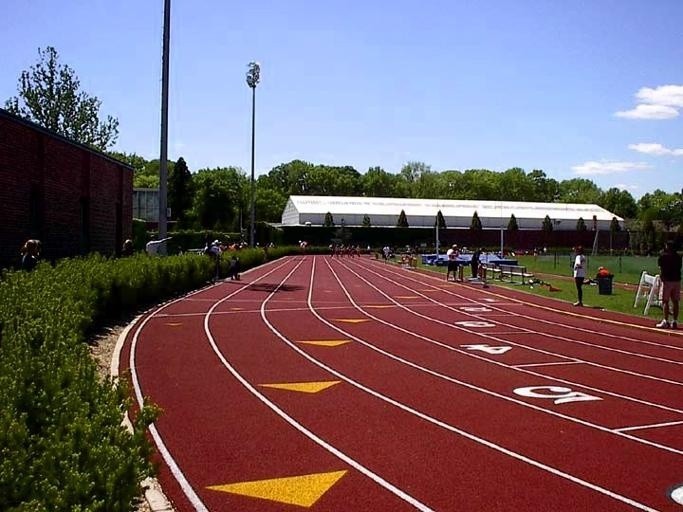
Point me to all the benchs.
[477,263,535,286]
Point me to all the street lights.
[241,56,261,250]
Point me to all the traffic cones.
[547,285,561,293]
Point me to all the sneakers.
[655,318,677,331]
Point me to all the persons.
[230,255,239,280]
[204,238,248,258]
[297,240,306,255]
[381,243,391,266]
[327,241,360,259]
[571,245,586,306]
[121,235,132,258]
[366,244,370,253]
[446,243,460,282]
[654,240,682,329]
[470,247,485,278]
[19,238,41,273]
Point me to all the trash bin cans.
[596,273,614,295]
